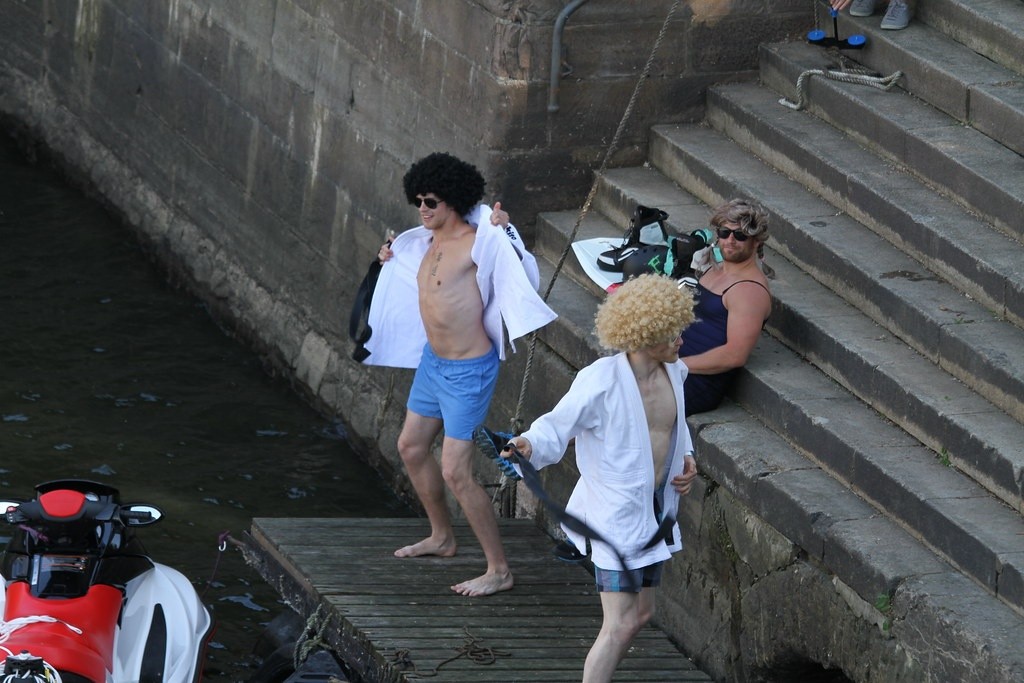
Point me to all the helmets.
[623,245,670,284]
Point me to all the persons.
[500,274,699,683]
[676,198,770,418]
[830,0,915,29]
[348,151,561,596]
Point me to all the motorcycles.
[0,478,217,683]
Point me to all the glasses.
[414,197,445,209]
[716,225,750,241]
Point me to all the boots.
[596,205,668,271]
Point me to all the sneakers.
[474,425,523,480]
[555,537,594,563]
[849,0,875,17]
[881,0,917,30]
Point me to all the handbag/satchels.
[672,229,712,281]
